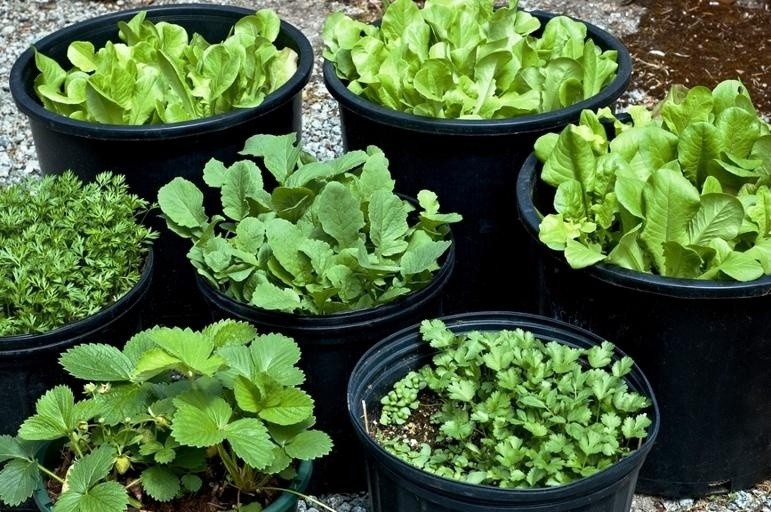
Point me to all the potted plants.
[316,1,634,313]
[515,77,770,498]
[346,310,662,511]
[0,168,170,511]
[0,315,339,512]
[152,129,465,496]
[9,4,312,331]
